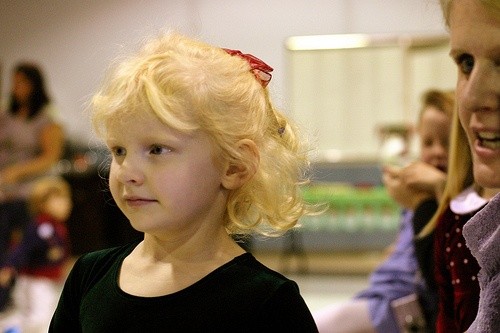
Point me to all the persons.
[47,39,321,333]
[315,0,500,333]
[0,63,72,333]
